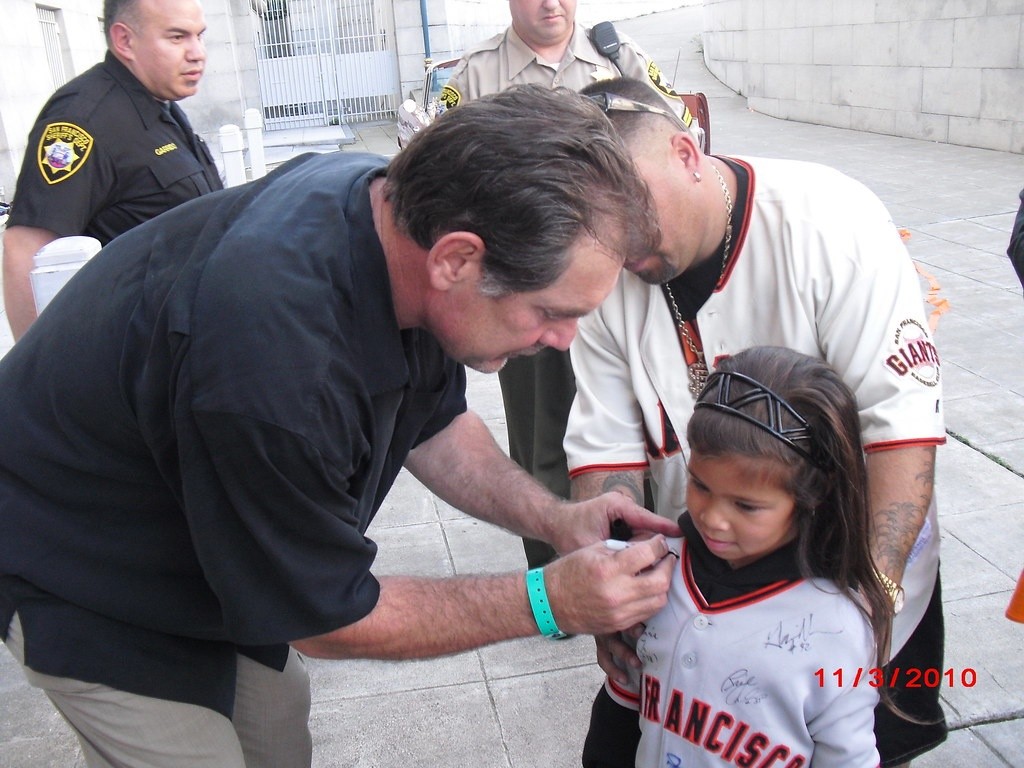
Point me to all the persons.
[435,0,691,637]
[564,77,950,768]
[2,0,225,345]
[582,347,893,768]
[0,85,684,768]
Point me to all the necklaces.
[666,165,733,397]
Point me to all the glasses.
[589,91,690,132]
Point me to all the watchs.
[871,568,905,617]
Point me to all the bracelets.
[526,567,576,639]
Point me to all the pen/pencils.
[605,538,678,559]
